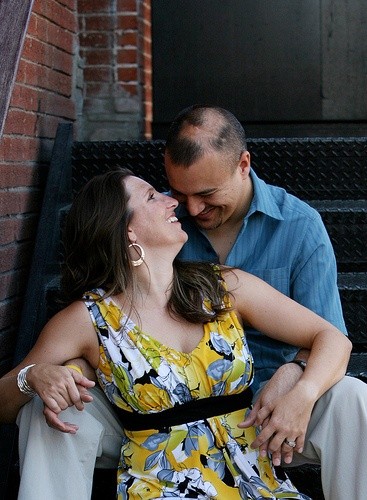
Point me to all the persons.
[15,103,367,500]
[0,167,353,500]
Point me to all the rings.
[284,440,297,447]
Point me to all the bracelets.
[286,359,307,372]
[16,364,38,398]
[65,365,82,375]
